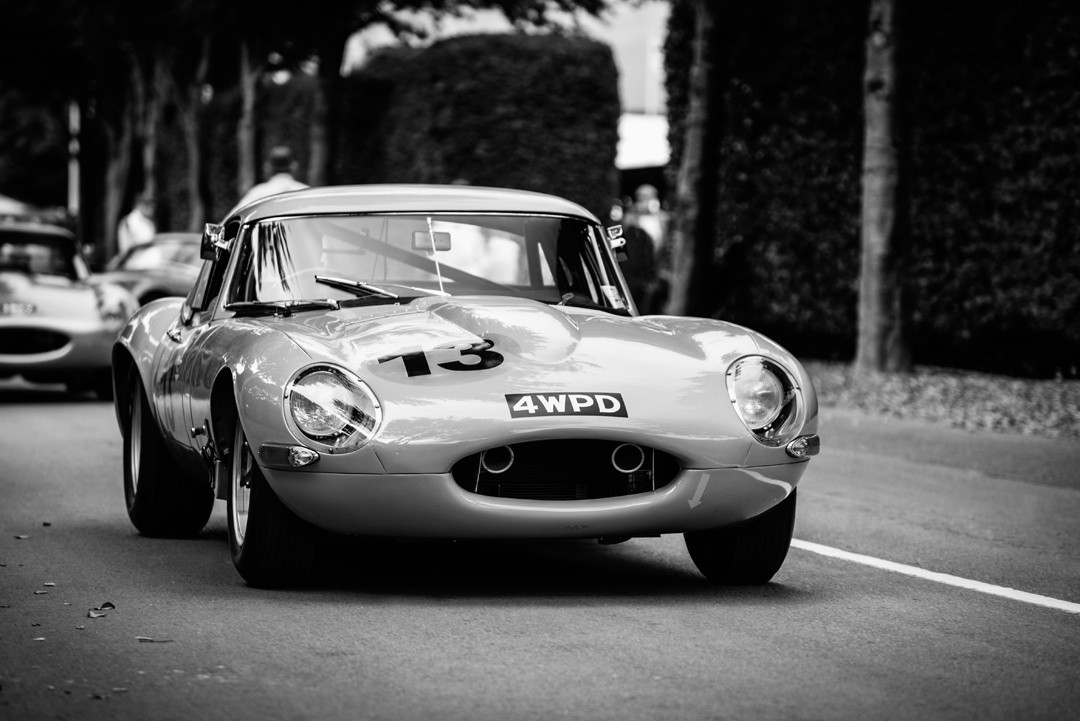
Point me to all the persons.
[206,226,321,317]
[612,185,669,315]
[238,144,310,205]
[117,192,157,254]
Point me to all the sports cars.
[0,209,203,396]
[112,183,819,592]
